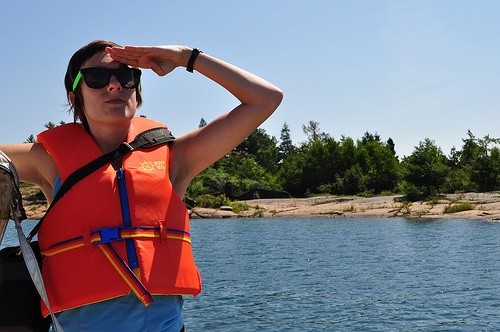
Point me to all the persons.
[0,38,284,332]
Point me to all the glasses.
[72,66,142,92]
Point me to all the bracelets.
[186,47,203,73]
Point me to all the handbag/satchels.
[0,241,50,332]
